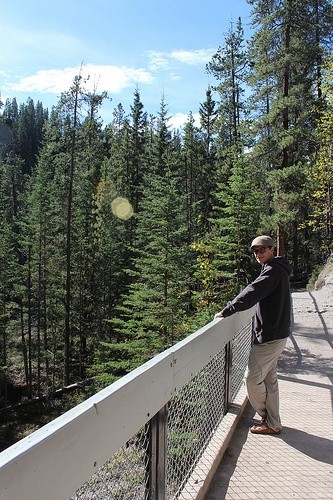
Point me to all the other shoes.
[250,423,281,434]
[261,417,266,425]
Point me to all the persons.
[214,235,290,434]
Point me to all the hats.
[251,236,274,249]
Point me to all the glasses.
[252,247,272,254]
